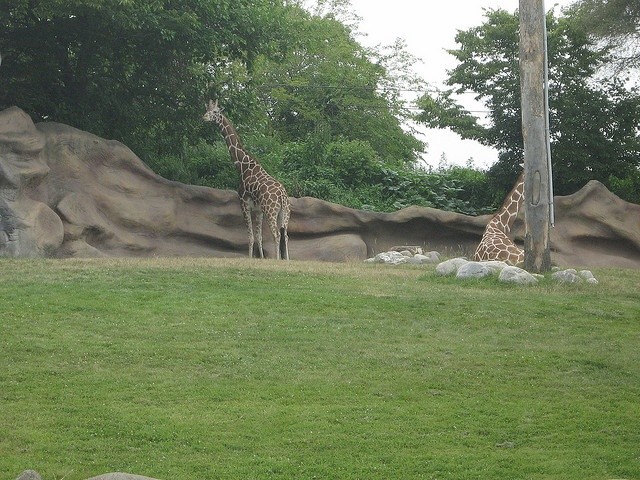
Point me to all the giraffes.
[474,163,526,269]
[201,98,292,262]
